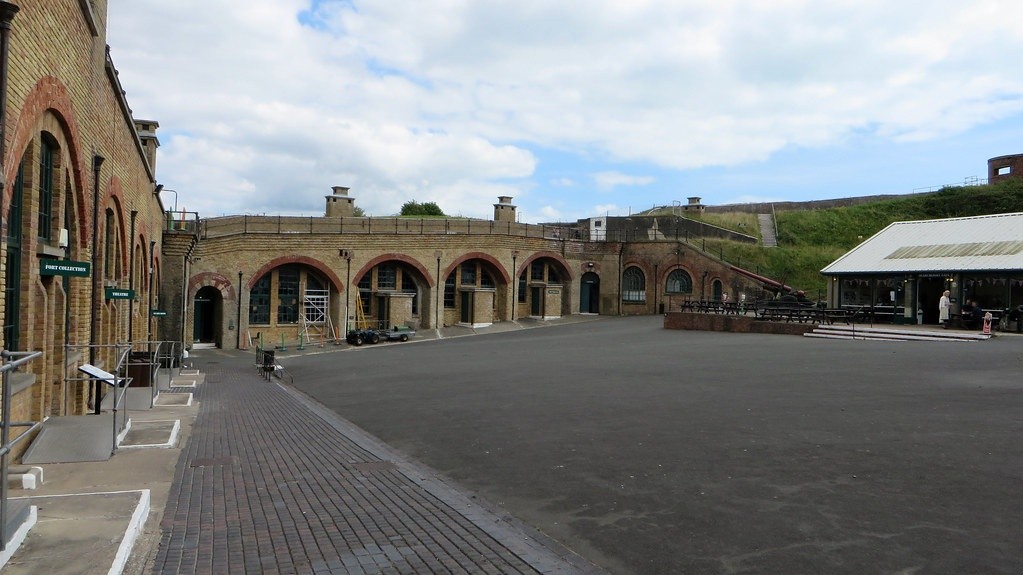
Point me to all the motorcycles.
[346,326,381,346]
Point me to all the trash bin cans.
[256,344,274,372]
[917,310,923,325]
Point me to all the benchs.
[680,305,874,318]
[942,319,973,322]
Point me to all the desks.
[709,301,722,314]
[849,308,871,323]
[743,303,757,316]
[727,302,738,315]
[682,300,699,312]
[763,307,793,323]
[794,309,821,323]
[823,309,850,325]
[945,314,970,329]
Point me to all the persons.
[721,292,728,305]
[938,291,952,329]
[966,300,982,311]
[183,347,189,368]
[739,293,746,312]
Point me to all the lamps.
[154,185,163,194]
